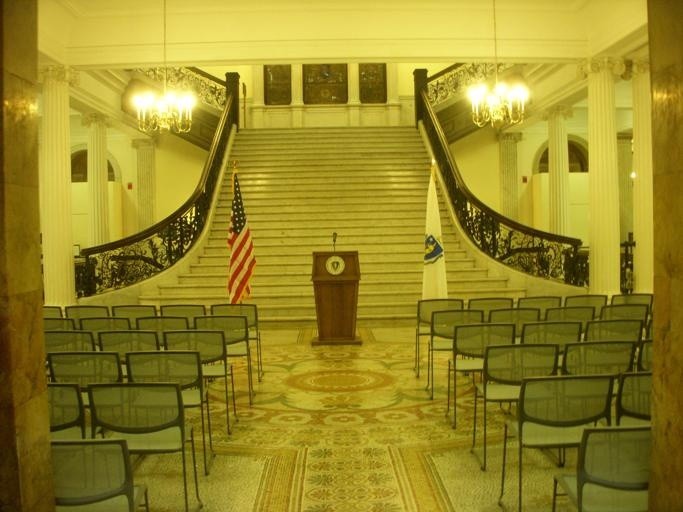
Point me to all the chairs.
[469,293,654,409]
[89,381,204,510]
[446,322,517,430]
[50,438,151,511]
[45,379,88,440]
[124,349,216,477]
[614,372,655,426]
[561,342,636,466]
[424,309,486,400]
[195,314,255,407]
[165,329,240,436]
[498,374,612,512]
[413,297,465,379]
[44,303,208,407]
[549,424,654,510]
[210,303,262,384]
[470,344,560,468]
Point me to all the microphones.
[333,232,337,242]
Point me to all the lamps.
[466,0,529,128]
[127,1,198,135]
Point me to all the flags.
[420,173,449,325]
[224,171,256,306]
